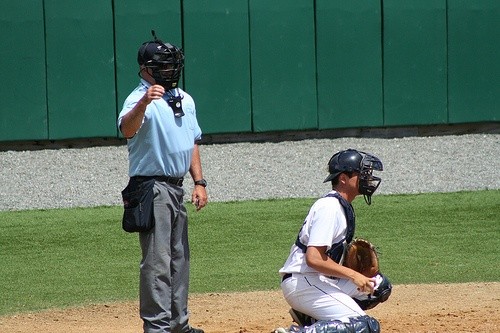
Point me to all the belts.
[282,273,292,281]
[161,177,186,187]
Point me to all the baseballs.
[366,281,376,292]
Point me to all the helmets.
[324,150,363,183]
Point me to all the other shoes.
[189,328,204,333]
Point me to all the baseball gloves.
[345,238,380,278]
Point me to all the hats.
[137,41,159,64]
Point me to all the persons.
[118,31,207,333]
[274,148,392,333]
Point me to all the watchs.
[193,179,207,188]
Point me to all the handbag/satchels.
[120,176,153,232]
